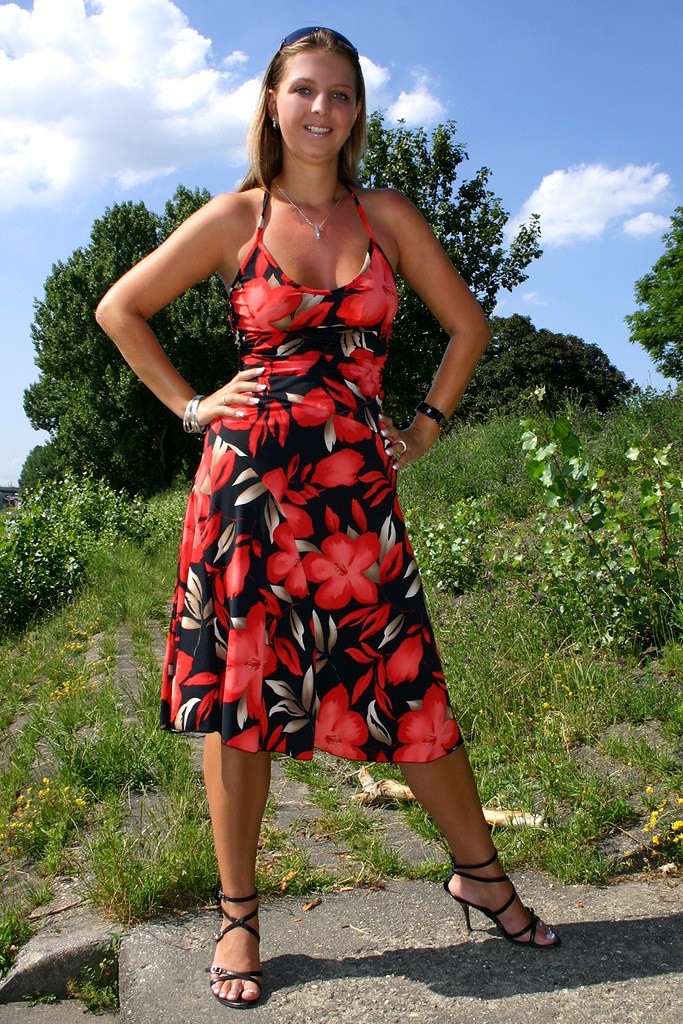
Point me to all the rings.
[222,394,228,406]
[395,441,406,452]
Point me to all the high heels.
[444,851,563,948]
[208,874,265,1009]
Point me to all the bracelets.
[183,395,209,434]
[415,401,449,433]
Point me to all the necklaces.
[272,179,347,239]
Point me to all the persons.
[94,29,560,1007]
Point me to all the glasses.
[277,25,359,61]
